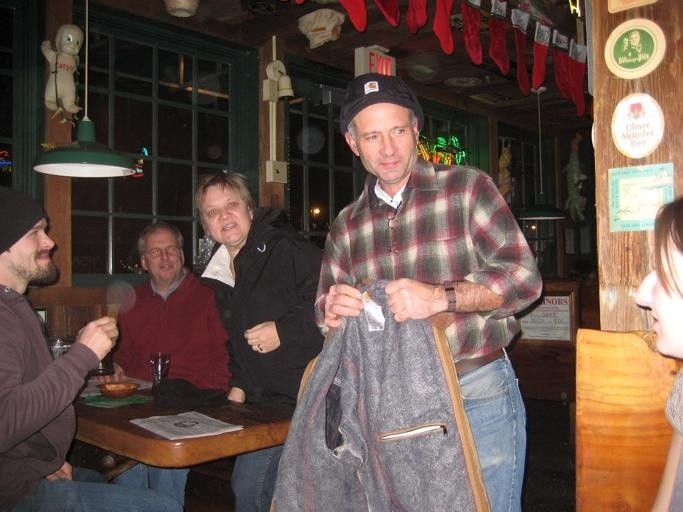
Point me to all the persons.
[314,71,543,511]
[634,195,683,512]
[195,169,325,511]
[0,188,185,512]
[109,220,233,511]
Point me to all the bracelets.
[441,280,456,312]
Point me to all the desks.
[72,372,298,469]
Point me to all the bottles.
[48,335,76,360]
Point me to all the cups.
[148,353,169,393]
[88,352,115,376]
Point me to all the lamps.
[32,0,136,180]
[516,85,569,221]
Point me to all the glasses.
[146,246,181,259]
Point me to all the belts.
[454,349,504,377]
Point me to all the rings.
[257,344,263,352]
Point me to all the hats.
[338,72,425,134]
[1,185,47,255]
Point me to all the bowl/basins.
[96,383,140,399]
